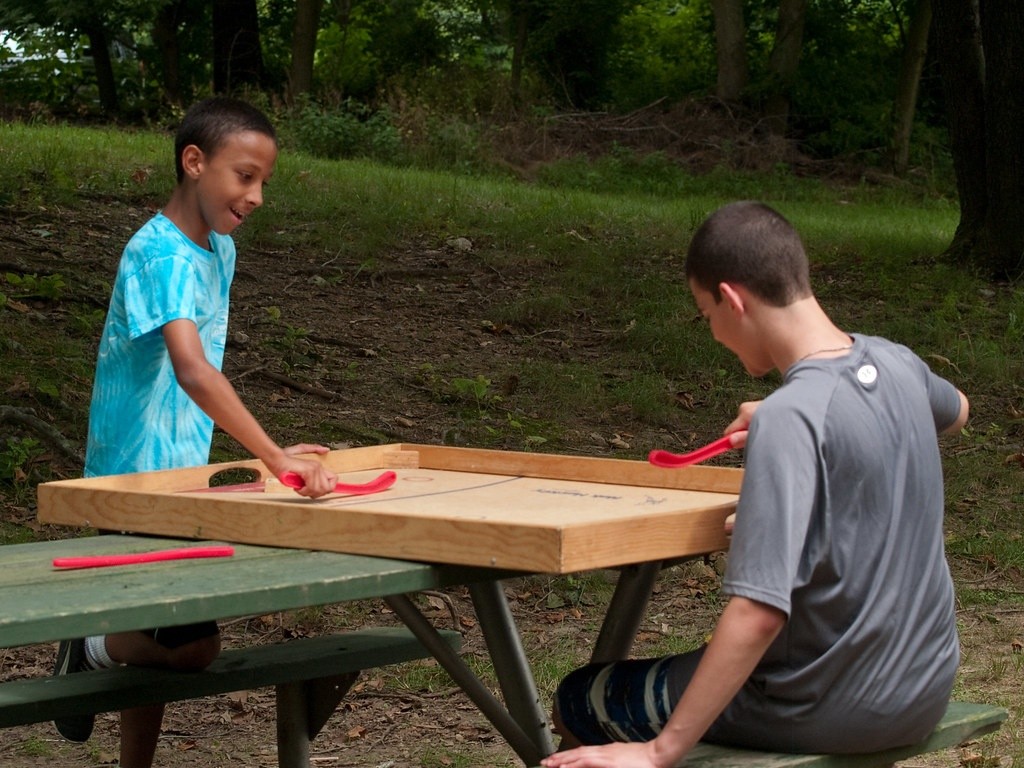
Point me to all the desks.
[0,531,717,768]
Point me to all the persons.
[50,97,338,768]
[538,200,970,768]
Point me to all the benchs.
[0,624,466,730]
[673,702,1014,768]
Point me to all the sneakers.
[51,637,95,744]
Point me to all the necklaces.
[798,345,852,361]
